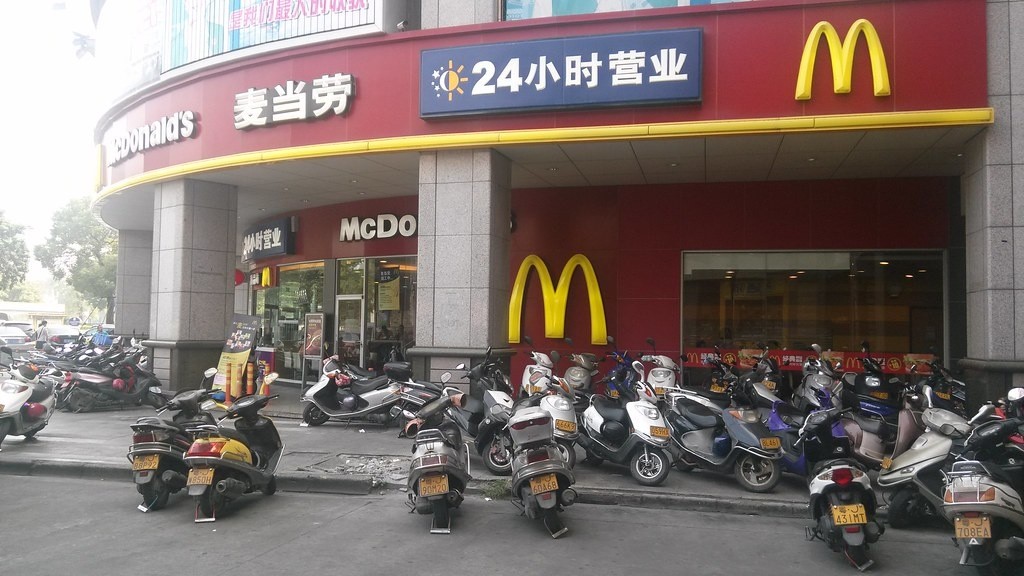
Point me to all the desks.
[367,340,404,355]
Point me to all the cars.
[0,323,115,348]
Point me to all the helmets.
[25,337,31,342]
[112,379,125,392]
[23,401,47,422]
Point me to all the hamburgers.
[310,330,321,348]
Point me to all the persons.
[338,336,345,346]
[90,324,109,341]
[379,325,393,339]
[394,325,407,338]
[36,320,48,349]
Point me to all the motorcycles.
[0,329,1024,576]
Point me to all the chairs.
[283,352,320,382]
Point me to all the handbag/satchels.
[36,341,47,348]
